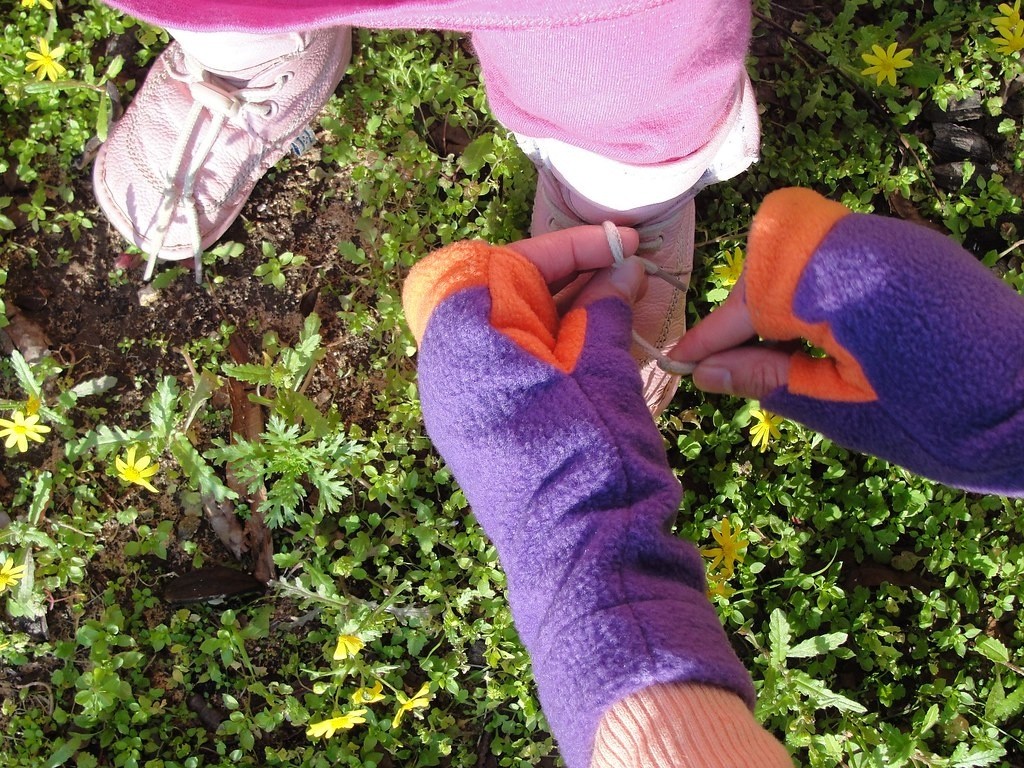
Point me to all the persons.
[92,0,761,418]
[402,187,1024,768]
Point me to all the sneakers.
[94,22,351,284]
[529,183,699,419]
